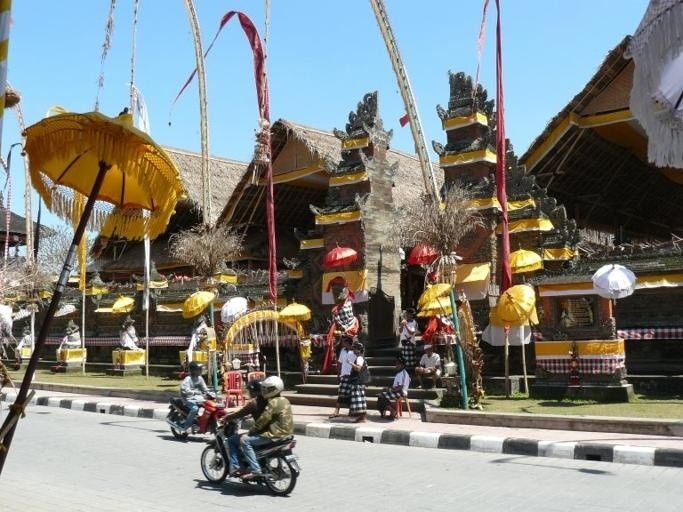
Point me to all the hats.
[353,342,365,354]
[423,344,433,350]
[341,334,353,346]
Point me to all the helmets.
[245,380,263,398]
[261,374,285,400]
[188,360,204,377]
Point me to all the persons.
[238,375,295,480]
[321,276,441,424]
[218,377,267,477]
[180,360,217,432]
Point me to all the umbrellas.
[509,240,545,282]
[497,284,553,340]
[620,1,683,172]
[407,241,438,269]
[277,296,313,383]
[324,245,358,271]
[421,296,456,317]
[590,261,638,301]
[415,282,453,317]
[111,294,135,315]
[0,104,187,474]
[51,303,76,318]
[219,295,248,324]
[182,290,222,346]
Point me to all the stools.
[383,397,412,420]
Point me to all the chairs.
[224,371,245,408]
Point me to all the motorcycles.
[198,416,302,497]
[164,392,231,441]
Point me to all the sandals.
[231,468,261,480]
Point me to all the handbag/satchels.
[350,357,370,385]
